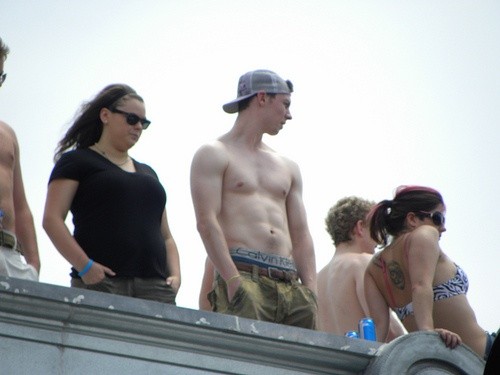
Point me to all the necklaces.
[95,142,130,167]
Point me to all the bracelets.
[225,273,241,285]
[78,258,94,277]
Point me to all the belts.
[233,261,299,282]
[0,231,22,256]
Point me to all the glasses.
[0,71,7,83]
[410,210,445,227]
[107,105,151,129]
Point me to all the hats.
[223,69,291,114]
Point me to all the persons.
[189,69,317,333]
[42,84,181,307]
[365,186,500,362]
[0,39,40,284]
[316,197,462,350]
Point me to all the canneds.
[344,331,360,338]
[358,316,377,341]
